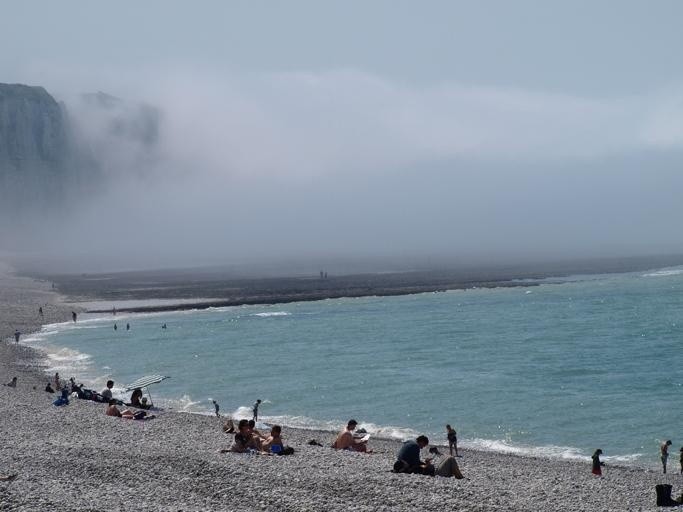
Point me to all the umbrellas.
[123,375,170,405]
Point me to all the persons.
[37,307,44,316]
[47,372,152,419]
[391,422,465,480]
[679,447,683,474]
[251,398,261,421]
[7,376,18,387]
[590,448,605,475]
[72,310,78,323]
[334,419,367,452]
[228,419,285,453]
[212,399,222,418]
[660,440,672,472]
[13,330,21,342]
